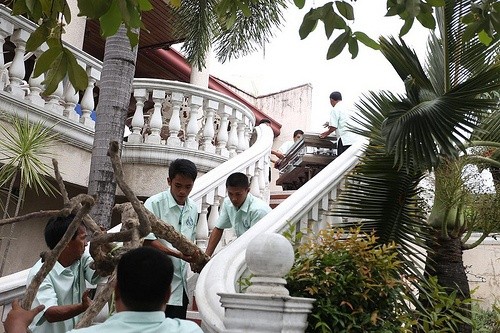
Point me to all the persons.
[278,130,304,159]
[321,91,359,158]
[24,215,124,333]
[258,118,273,182]
[4,246,205,333]
[206,171,272,258]
[142,159,198,320]
